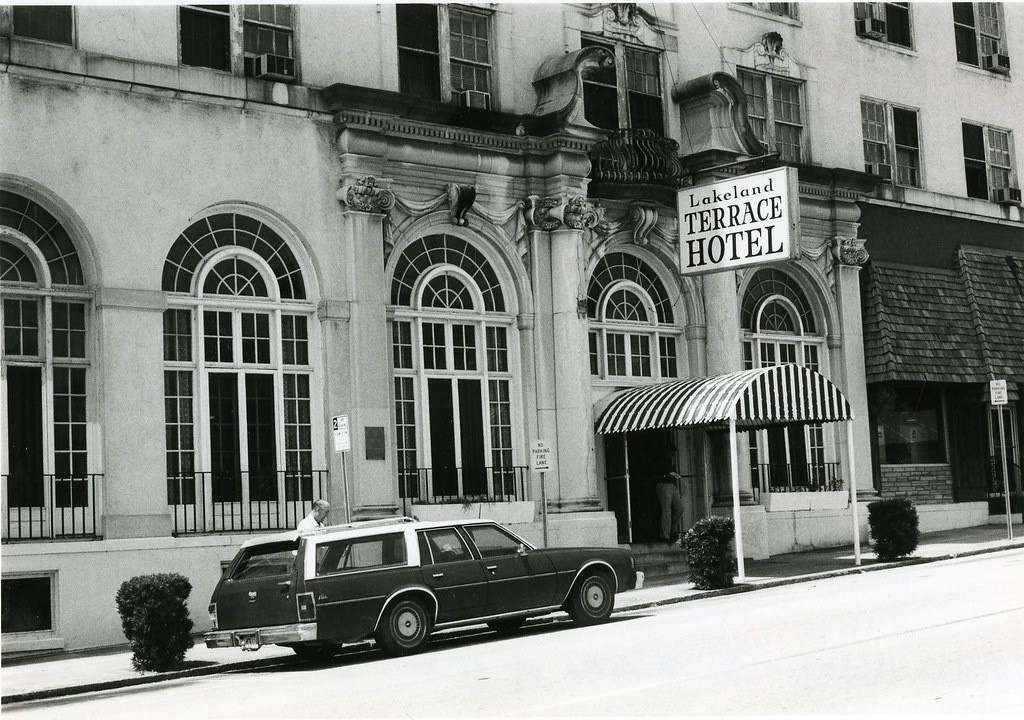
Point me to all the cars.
[203,514,645,665]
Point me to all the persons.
[652,441,686,545]
[288,499,333,577]
